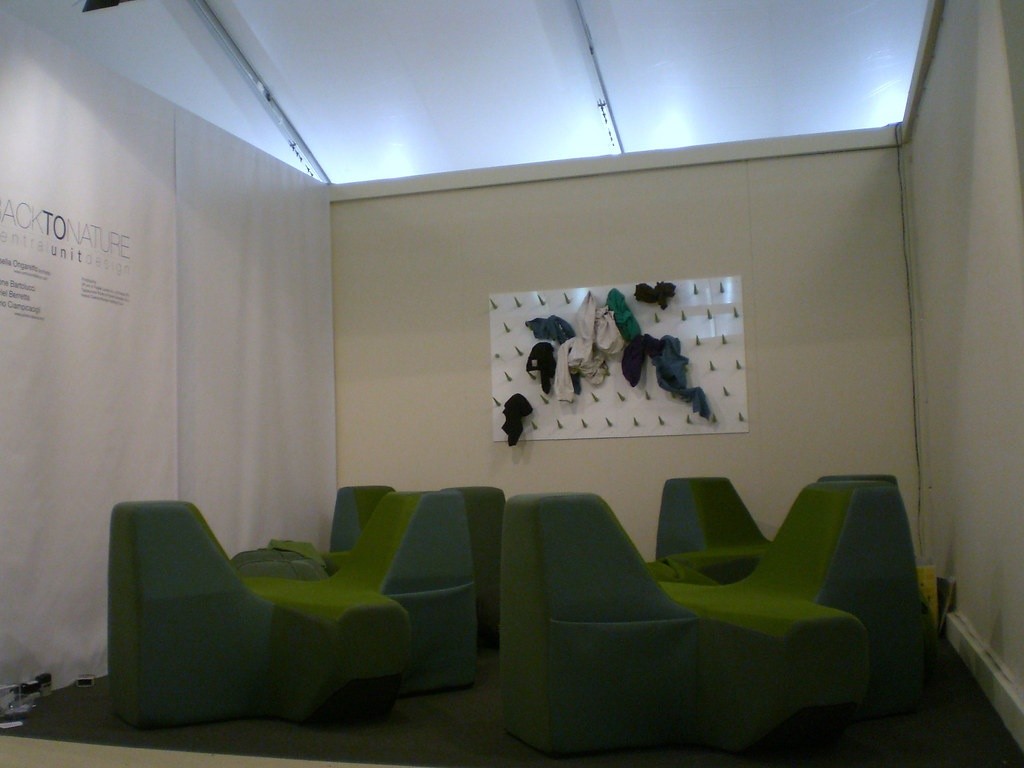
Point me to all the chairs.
[107,475,926,760]
